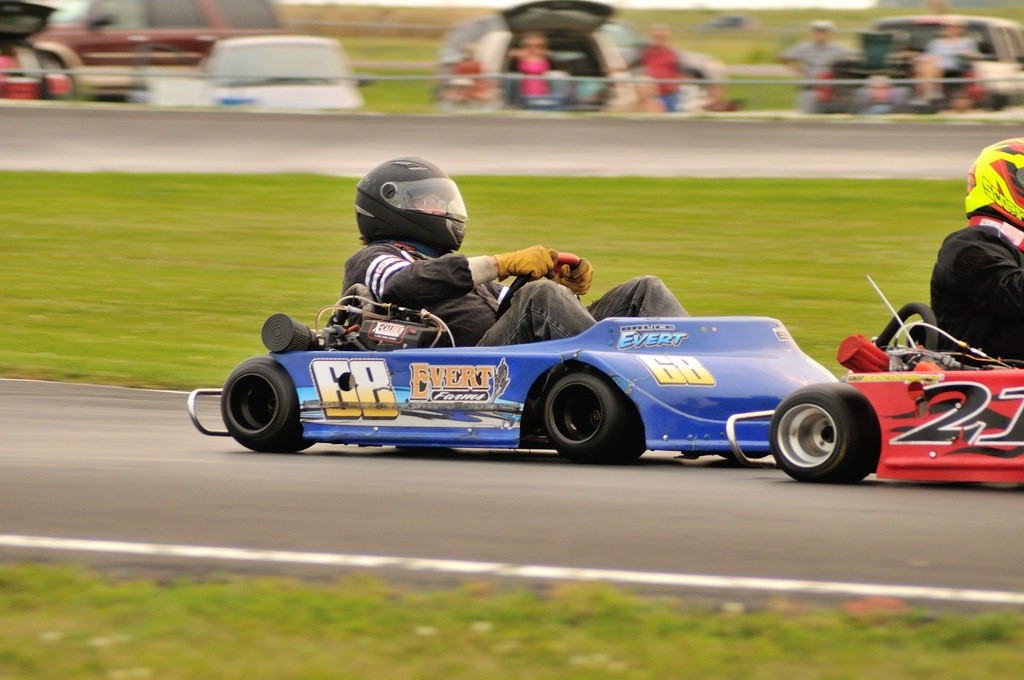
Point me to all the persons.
[929,138,1024,364]
[602,25,708,112]
[340,157,690,350]
[448,45,484,97]
[510,33,553,97]
[861,20,981,112]
[781,20,856,112]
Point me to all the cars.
[427,0,1024,117]
[1,1,361,107]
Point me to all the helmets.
[965,137,1024,228]
[355,157,468,251]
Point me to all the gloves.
[494,244,558,282]
[554,258,594,295]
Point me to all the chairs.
[908,326,944,352]
